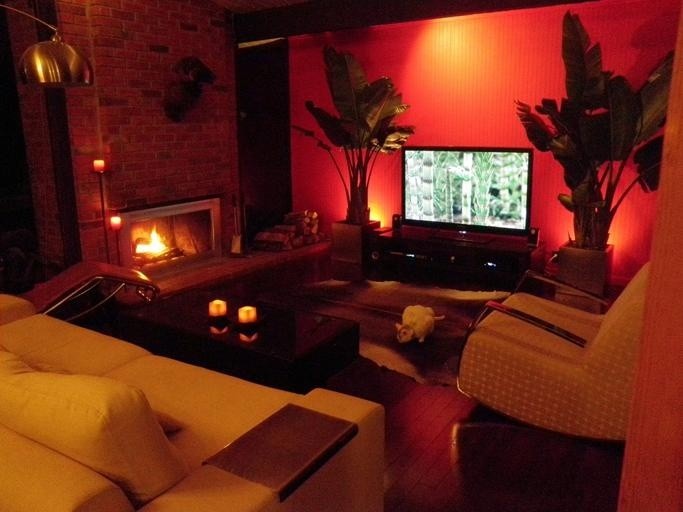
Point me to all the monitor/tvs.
[401,144,534,245]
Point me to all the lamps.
[1,1,92,86]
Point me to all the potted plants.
[279,44,416,279]
[513,11,675,295]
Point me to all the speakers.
[527,226,539,247]
[392,214,402,230]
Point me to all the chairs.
[457,260,650,442]
[19,262,161,321]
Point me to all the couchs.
[0,294,385,512]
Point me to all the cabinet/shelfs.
[377,225,546,290]
